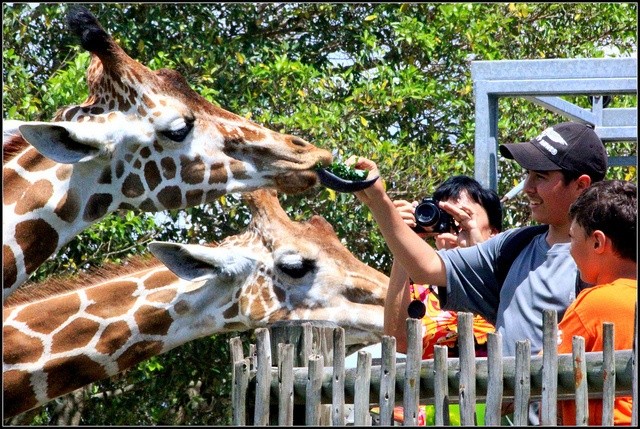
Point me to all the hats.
[499,121,608,181]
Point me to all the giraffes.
[2,4,334,304]
[3,187,392,421]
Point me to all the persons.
[342,122,607,428]
[384,176,502,358]
[535,181,637,427]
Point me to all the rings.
[457,218,464,223]
